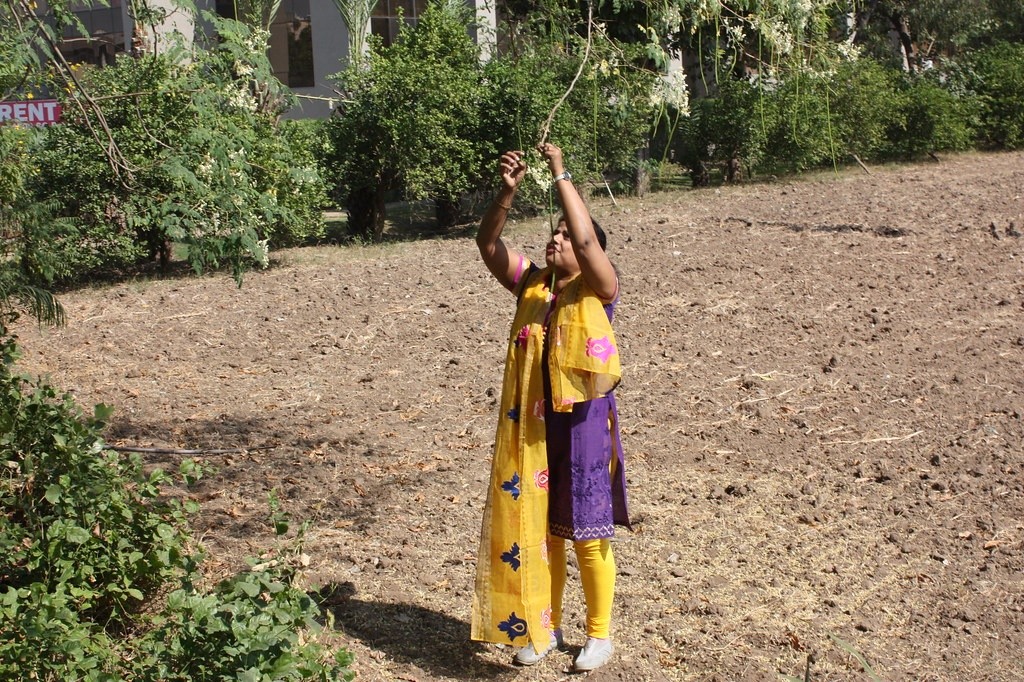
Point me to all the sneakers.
[513,633,564,665]
[572,639,612,671]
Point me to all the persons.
[471,143,634,672]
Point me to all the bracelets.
[496,197,511,210]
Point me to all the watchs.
[553,172,572,183]
